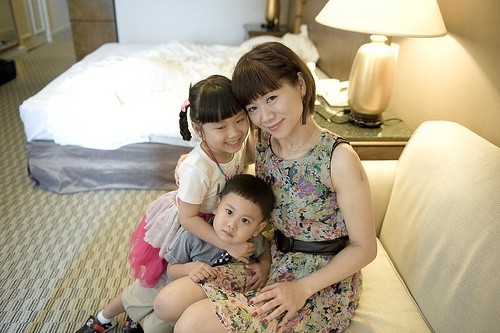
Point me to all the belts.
[272,229,348,255]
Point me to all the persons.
[153,42,378,333]
[74,174,276,333]
[128,76,257,288]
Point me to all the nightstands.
[243,23,289,41]
[306,95,411,162]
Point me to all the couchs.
[344,120,500,333]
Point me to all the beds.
[18,42,330,198]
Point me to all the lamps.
[314,0,448,126]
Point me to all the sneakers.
[76,315,118,333]
[124,317,144,333]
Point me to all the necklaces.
[203,141,240,179]
[277,125,317,162]
